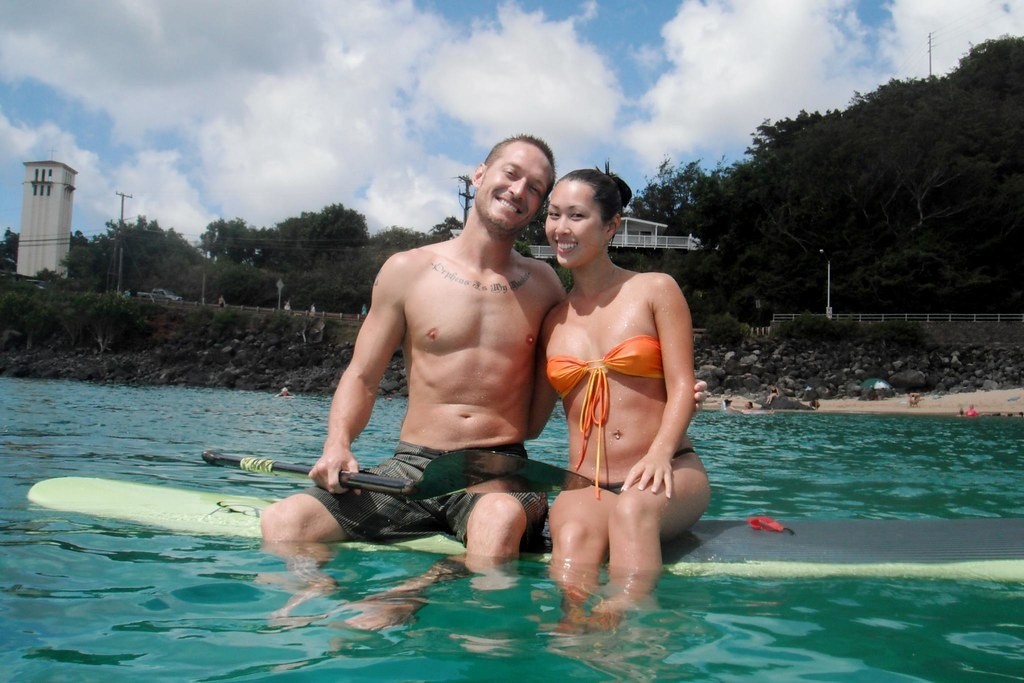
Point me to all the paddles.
[202,448,592,499]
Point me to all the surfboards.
[25,475,1024,584]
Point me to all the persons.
[725,377,1023,426]
[262,133,707,559]
[119,285,368,323]
[527,170,711,571]
[275,387,294,397]
